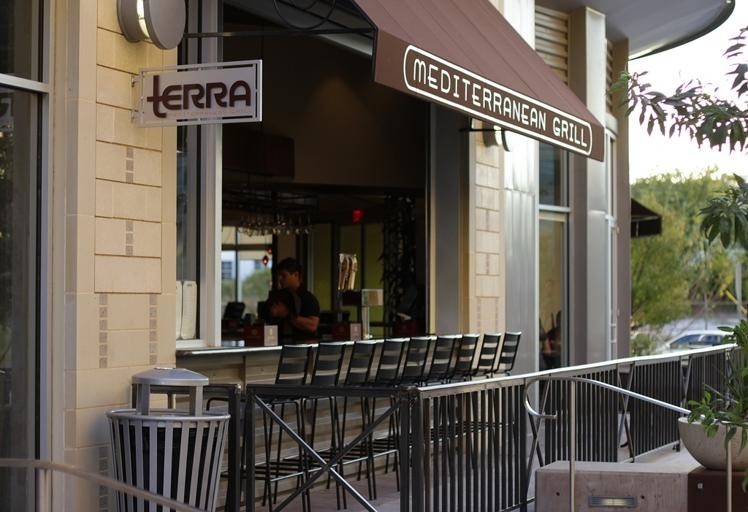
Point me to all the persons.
[260,257,321,345]
[545,311,562,368]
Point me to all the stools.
[429,336,480,479]
[310,339,377,509]
[383,337,432,492]
[397,335,457,479]
[206,344,311,511]
[446,332,502,473]
[339,338,405,500]
[408,336,455,477]
[260,342,347,511]
[473,330,521,470]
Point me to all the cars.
[646,327,738,359]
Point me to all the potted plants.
[670,319,748,471]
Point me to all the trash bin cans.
[105,368,232,512]
